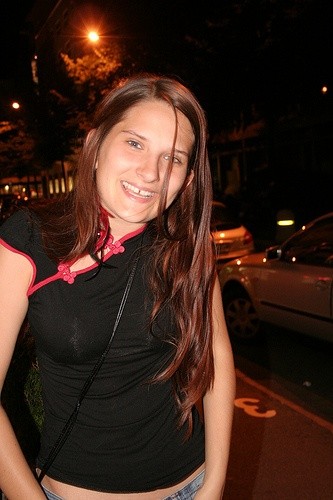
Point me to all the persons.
[0,76,237,500]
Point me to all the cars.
[209,202,333,347]
